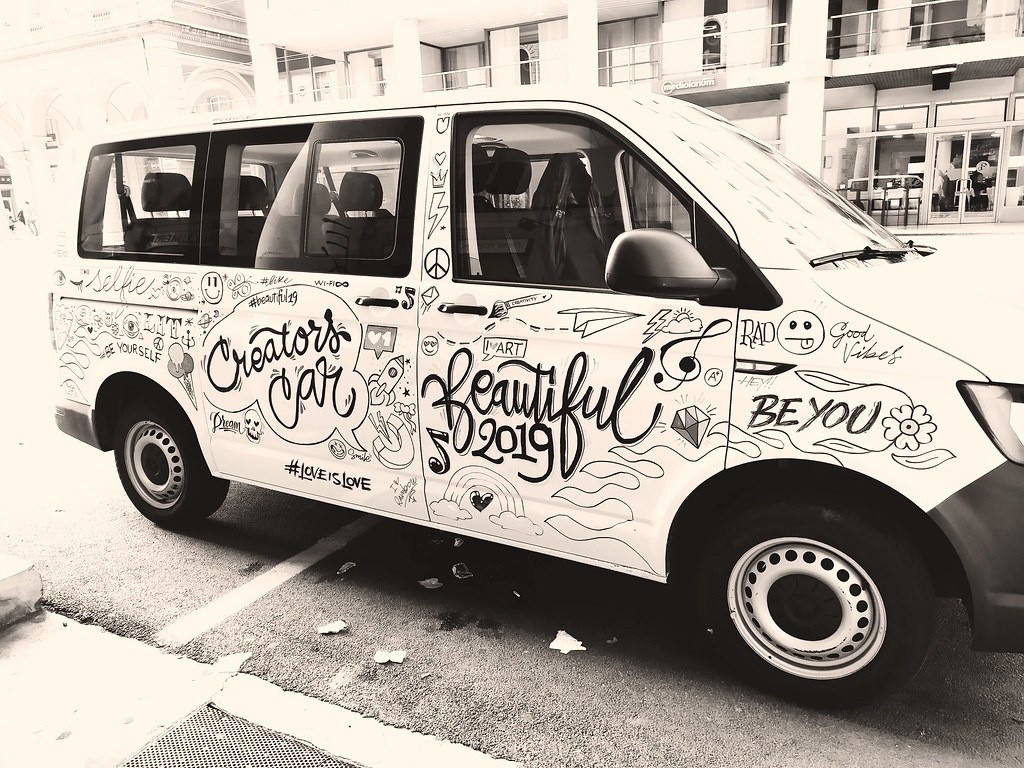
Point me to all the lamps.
[931,68,956,91]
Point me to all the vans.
[846,174,924,211]
[44,78,1023,715]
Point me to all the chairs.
[531,153,607,289]
[474,142,532,283]
[238,172,395,270]
[123,173,192,252]
